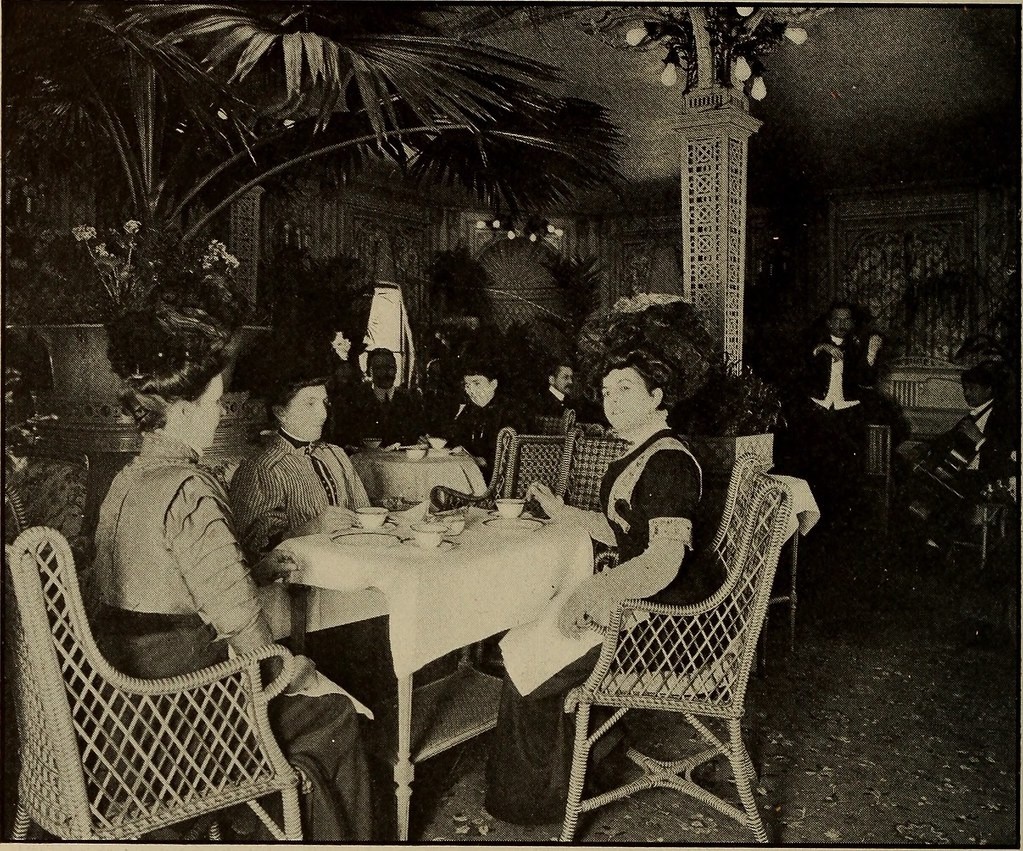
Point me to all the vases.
[6,323,273,421]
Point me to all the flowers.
[9,219,256,321]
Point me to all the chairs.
[0,408,1022,842]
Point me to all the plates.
[399,537,457,552]
[486,510,533,518]
[482,517,546,533]
[350,523,397,532]
[328,532,402,549]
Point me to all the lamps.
[626,8,804,101]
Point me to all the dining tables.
[349,447,485,503]
[266,504,593,840]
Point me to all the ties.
[381,393,391,414]
[555,399,570,406]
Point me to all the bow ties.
[833,342,846,352]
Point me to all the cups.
[441,516,466,536]
[405,450,426,460]
[363,438,382,448]
[408,522,449,547]
[355,507,389,529]
[495,499,525,518]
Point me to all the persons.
[95,333,375,842]
[895,365,1013,550]
[484,351,737,826]
[229,363,374,564]
[445,369,517,462]
[533,363,575,420]
[806,303,881,413]
[348,349,418,447]
